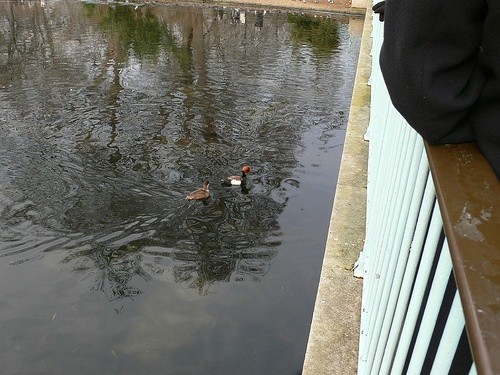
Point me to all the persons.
[371,0,500,185]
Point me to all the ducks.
[221,166,253,186]
[183,180,211,200]
